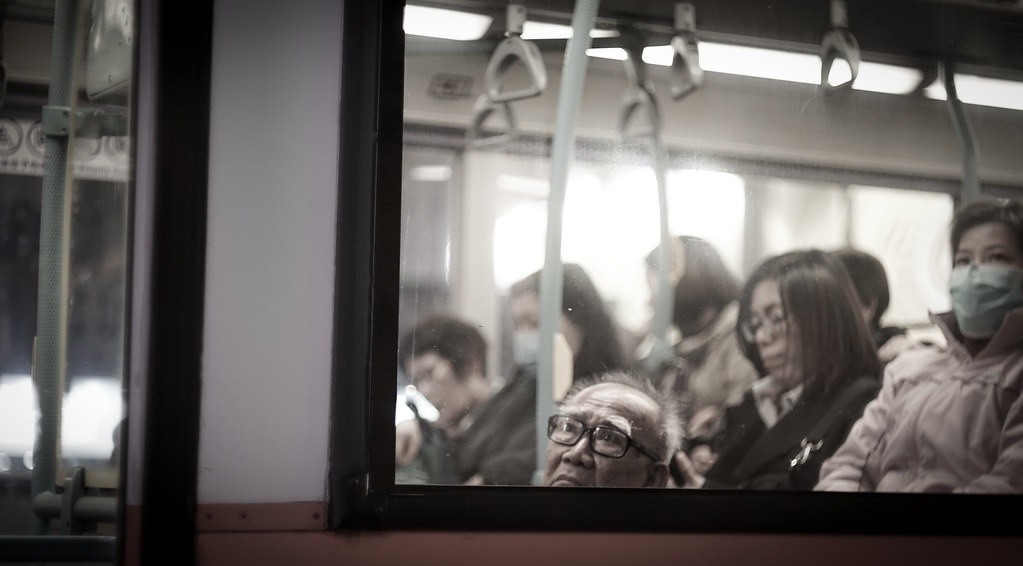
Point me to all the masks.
[512,329,538,380]
[949,262,1023,338]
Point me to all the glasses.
[740,307,787,341]
[547,414,661,462]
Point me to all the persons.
[399,234,906,493]
[813,197,1023,495]
